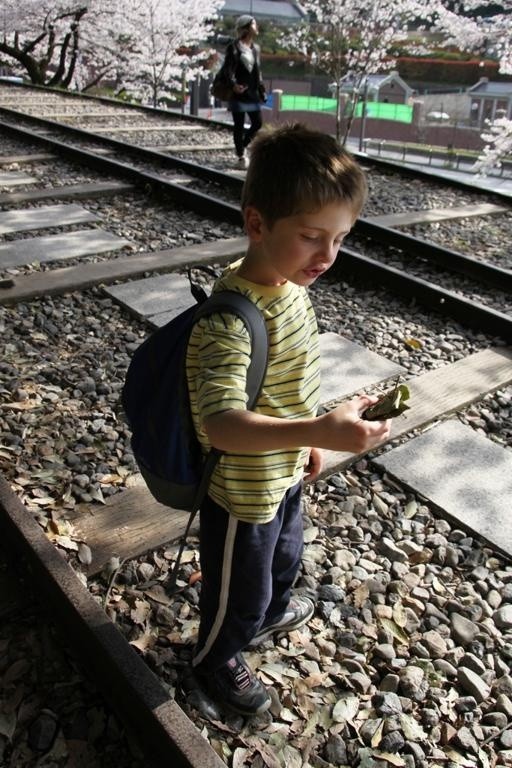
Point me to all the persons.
[224,14,269,171]
[182,125,394,717]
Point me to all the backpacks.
[118,264,269,512]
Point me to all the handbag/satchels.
[211,68,232,101]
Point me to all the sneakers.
[207,651,272,716]
[248,596,315,646]
[235,147,250,169]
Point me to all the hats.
[236,14,253,31]
